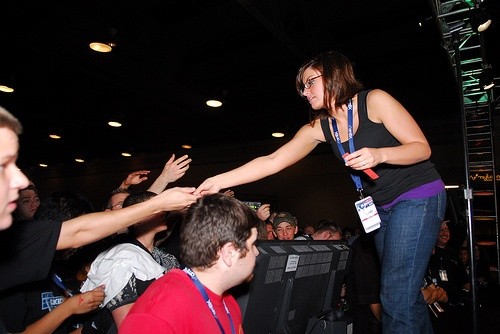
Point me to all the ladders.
[455,18,500,312]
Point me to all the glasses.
[109,201,125,209]
[303,74,322,89]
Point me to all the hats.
[272,212,296,228]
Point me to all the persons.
[421,220,470,334]
[456,247,470,274]
[345,227,448,334]
[472,244,489,321]
[0,106,202,334]
[192,56,447,334]
[263,209,360,298]
[118,192,259,334]
